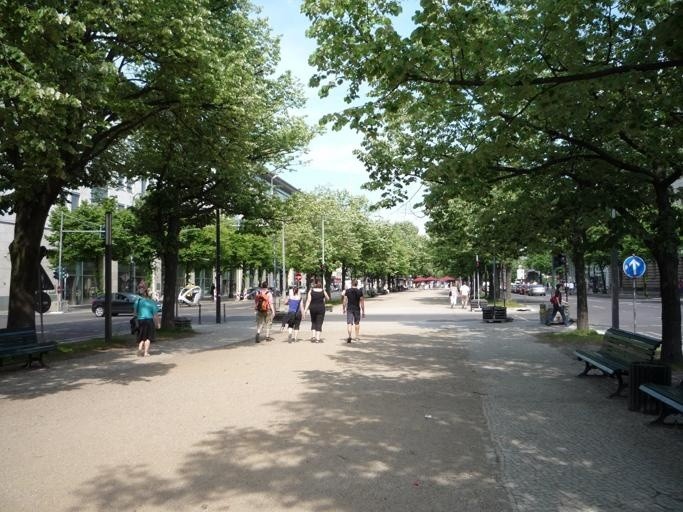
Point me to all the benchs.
[572,327,683,427]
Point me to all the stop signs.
[295,273,301,281]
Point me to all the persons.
[283,286,302,344]
[370,279,450,294]
[253,281,276,343]
[341,279,364,344]
[447,281,458,309]
[131,288,161,358]
[304,278,329,344]
[544,282,574,328]
[458,280,470,310]
[209,282,215,300]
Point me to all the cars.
[91,292,162,318]
[510,280,546,297]
[240,285,307,300]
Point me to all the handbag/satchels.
[548,294,559,305]
[449,291,452,297]
[129,315,140,336]
[286,311,296,325]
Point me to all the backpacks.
[254,290,271,312]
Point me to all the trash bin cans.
[629,362,671,414]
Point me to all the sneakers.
[254,332,361,344]
[136,348,151,359]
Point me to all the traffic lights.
[53,267,59,280]
